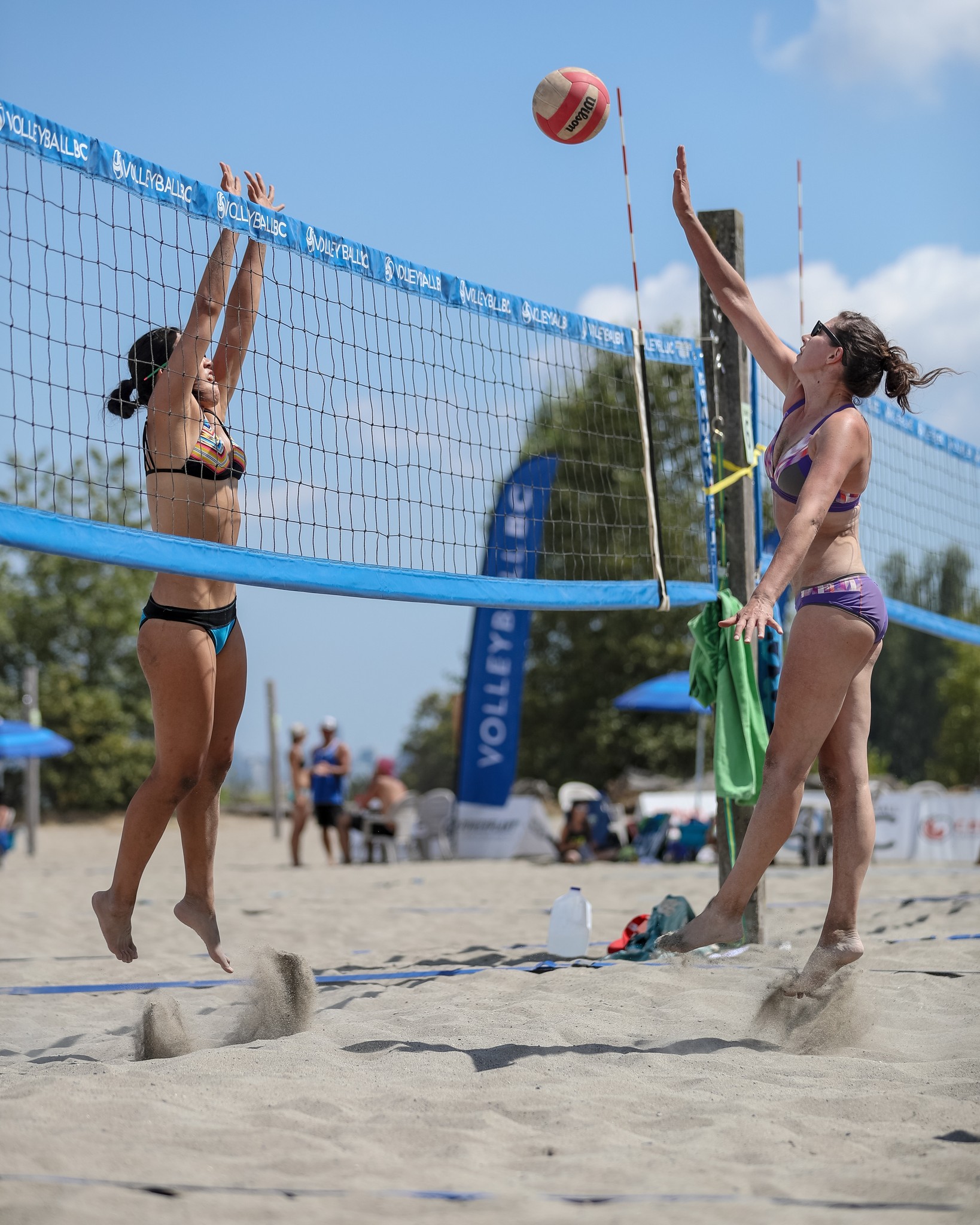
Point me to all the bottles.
[547,885,591,959]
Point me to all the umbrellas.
[613,669,714,821]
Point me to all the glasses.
[810,320,848,367]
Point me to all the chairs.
[365,790,428,863]
[416,787,456,862]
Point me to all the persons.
[561,785,619,864]
[0,790,17,855]
[0,715,76,761]
[90,161,287,976]
[655,144,937,995]
[286,718,409,868]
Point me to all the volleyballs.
[531,66,611,146]
